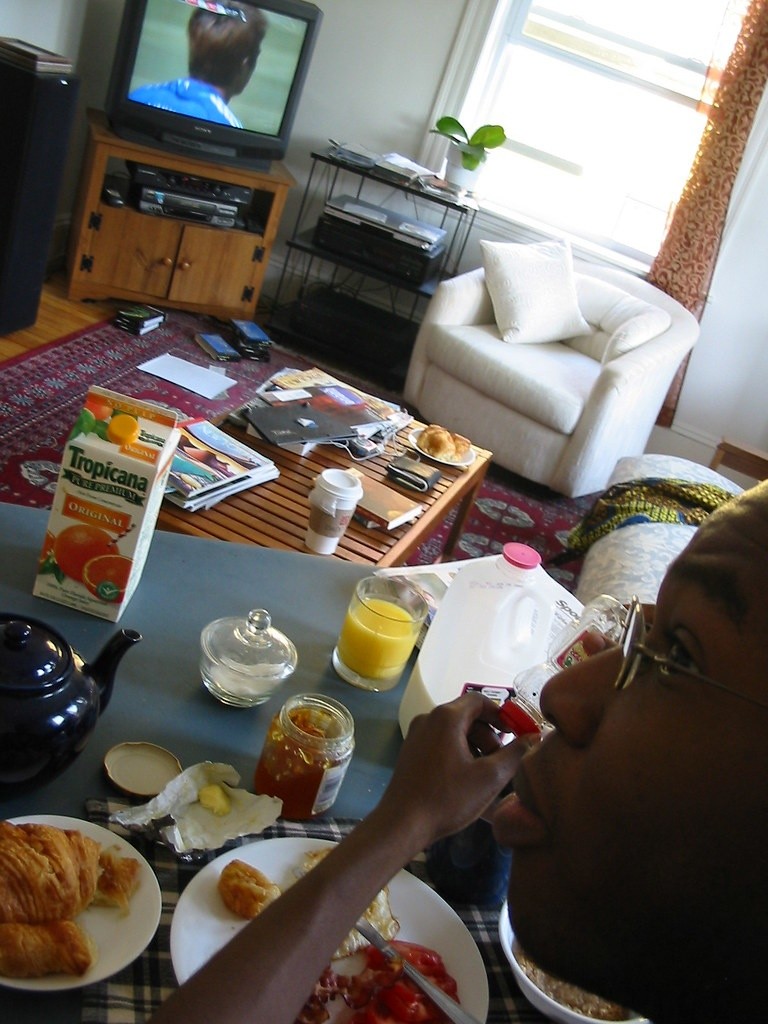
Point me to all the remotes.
[101,185,125,208]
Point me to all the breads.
[417,423,471,462]
[218,858,282,918]
[0,821,141,977]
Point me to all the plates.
[408,427,476,466]
[169,837,490,1024]
[0,814,163,991]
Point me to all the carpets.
[0,307,590,596]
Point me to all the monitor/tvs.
[105,0,325,174]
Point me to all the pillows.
[479,239,592,343]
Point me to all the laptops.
[324,194,448,252]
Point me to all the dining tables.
[1,502,552,1024]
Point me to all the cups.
[332,576,429,693]
[304,468,364,556]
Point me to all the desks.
[152,366,493,570]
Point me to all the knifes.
[292,865,484,1024]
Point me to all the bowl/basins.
[199,608,297,708]
[499,898,650,1024]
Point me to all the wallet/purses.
[388,457,442,492]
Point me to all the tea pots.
[0,610,143,799]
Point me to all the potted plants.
[429,116,508,193]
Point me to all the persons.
[126,0,269,129]
[146,479,768,1024]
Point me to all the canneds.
[255,692,356,823]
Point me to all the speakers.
[0,57,82,336]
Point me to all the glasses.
[613,596,768,708]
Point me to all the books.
[194,318,272,362]
[227,366,414,457]
[329,138,478,210]
[312,468,423,530]
[164,417,280,511]
[115,304,166,336]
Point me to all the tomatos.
[358,938,459,1024]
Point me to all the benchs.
[574,438,768,613]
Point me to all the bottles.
[498,592,629,739]
[398,541,556,741]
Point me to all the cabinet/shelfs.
[262,144,477,391]
[65,108,296,324]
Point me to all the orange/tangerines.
[40,524,134,603]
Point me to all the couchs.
[403,267,701,499]
